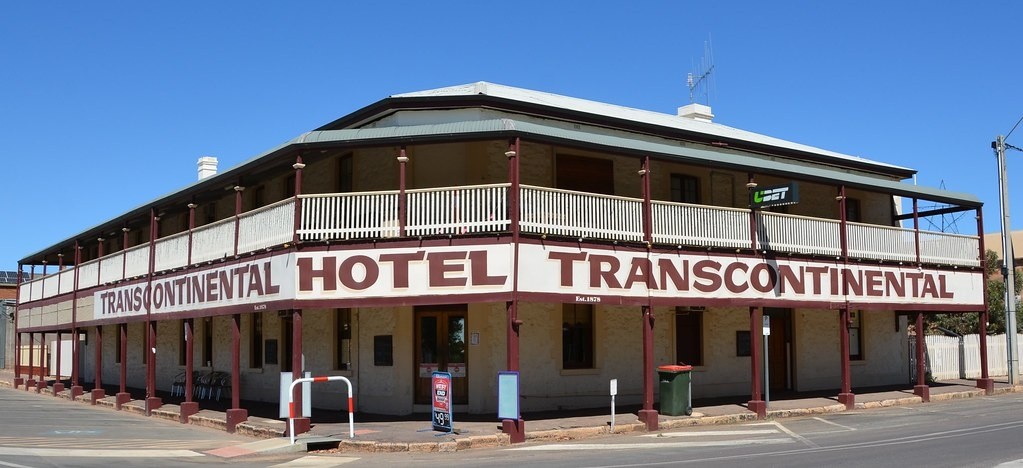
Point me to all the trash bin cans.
[655,364,693,417]
